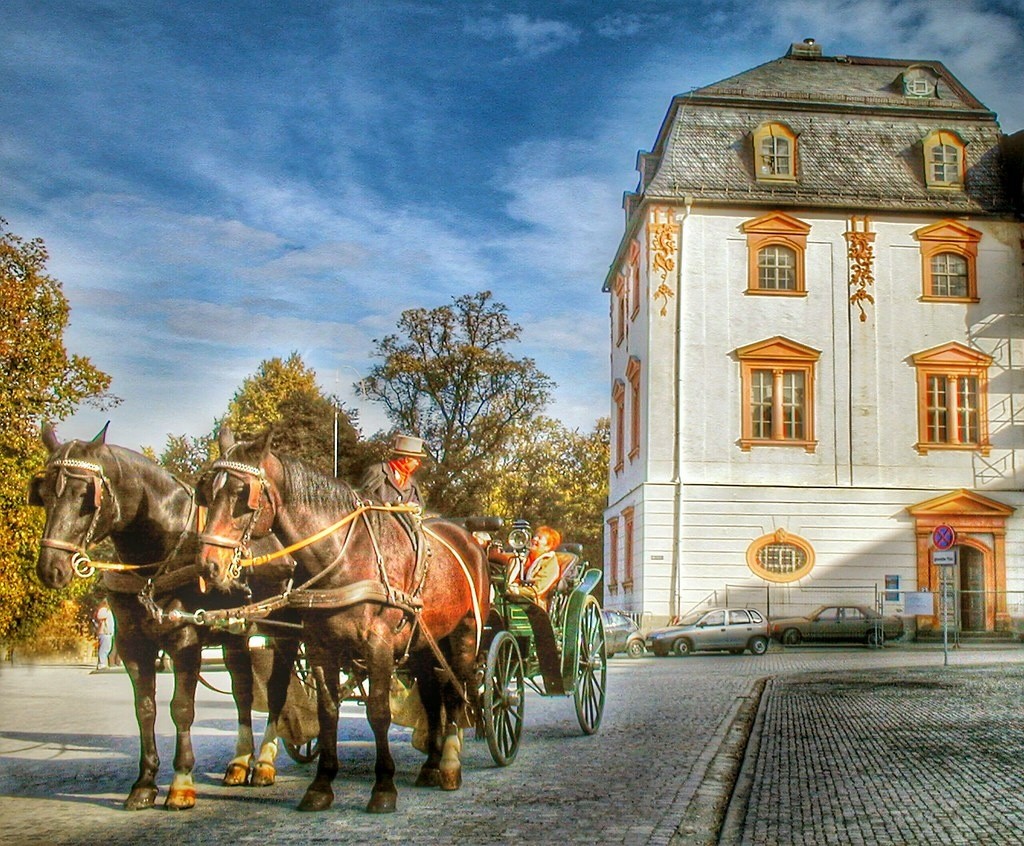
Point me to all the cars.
[644,607,773,655]
[768,602,902,649]
[155,636,267,673]
[582,609,646,659]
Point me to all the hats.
[388,434,428,458]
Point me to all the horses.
[26,420,300,812]
[193,419,491,812]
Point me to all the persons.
[508,526,560,612]
[94,599,114,670]
[357,435,427,512]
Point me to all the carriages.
[35,420,610,813]
